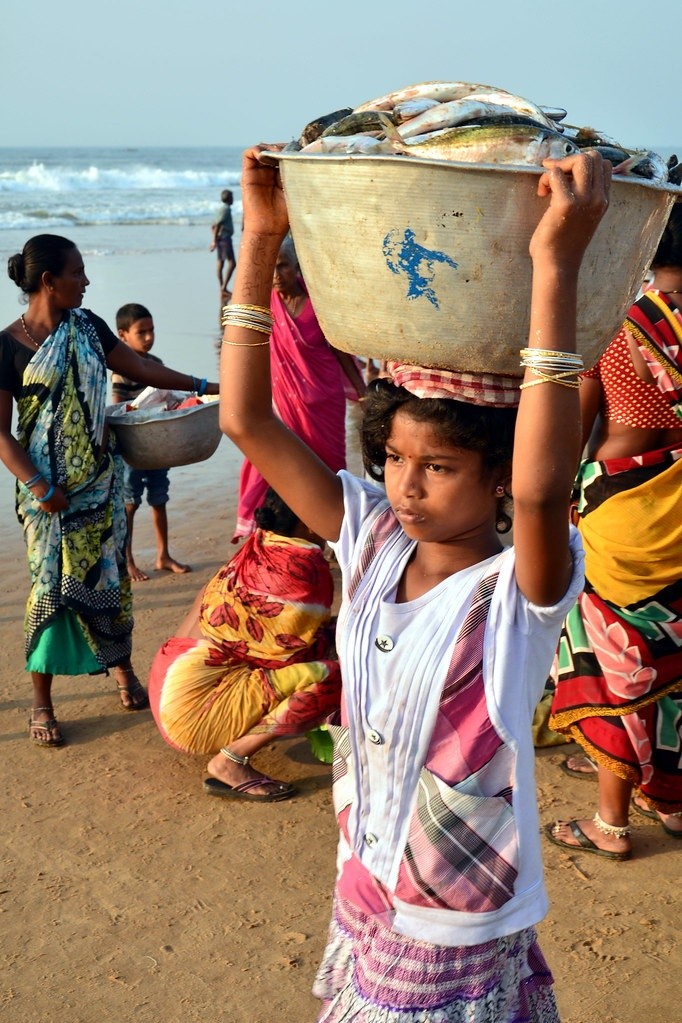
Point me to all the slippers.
[117,678,151,709]
[544,818,633,862]
[630,796,681,837]
[27,718,65,747]
[560,755,600,781]
[202,773,300,802]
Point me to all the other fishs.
[280,80,682,183]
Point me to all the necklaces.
[660,284,680,298]
[19,312,44,350]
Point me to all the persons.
[147,480,341,803]
[0,233,221,747]
[111,303,192,581]
[217,234,372,545]
[217,141,619,1023]
[559,748,608,780]
[545,183,681,862]
[208,189,239,298]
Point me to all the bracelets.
[190,374,196,395]
[198,378,207,397]
[26,474,43,489]
[220,301,273,336]
[24,473,41,485]
[220,338,271,347]
[357,394,371,402]
[511,345,585,392]
[34,485,55,502]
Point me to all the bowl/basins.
[105,393,224,472]
[258,148,681,375]
